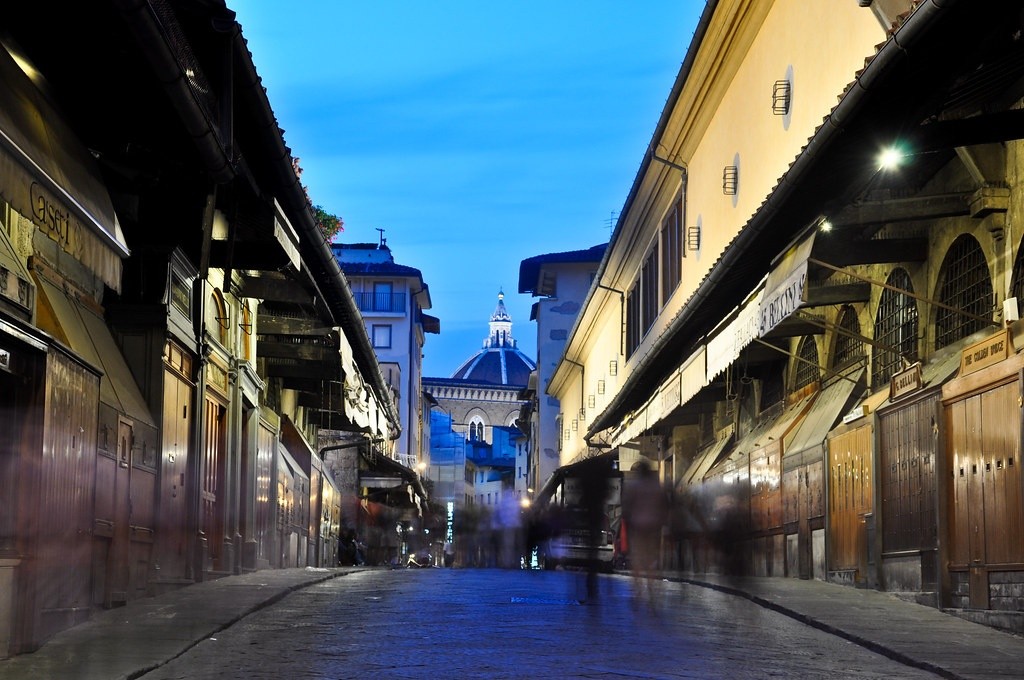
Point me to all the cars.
[543,507,616,572]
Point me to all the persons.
[350,534,455,569]
[625,462,758,618]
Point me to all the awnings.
[610,230,819,448]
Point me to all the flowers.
[311,205,345,247]
